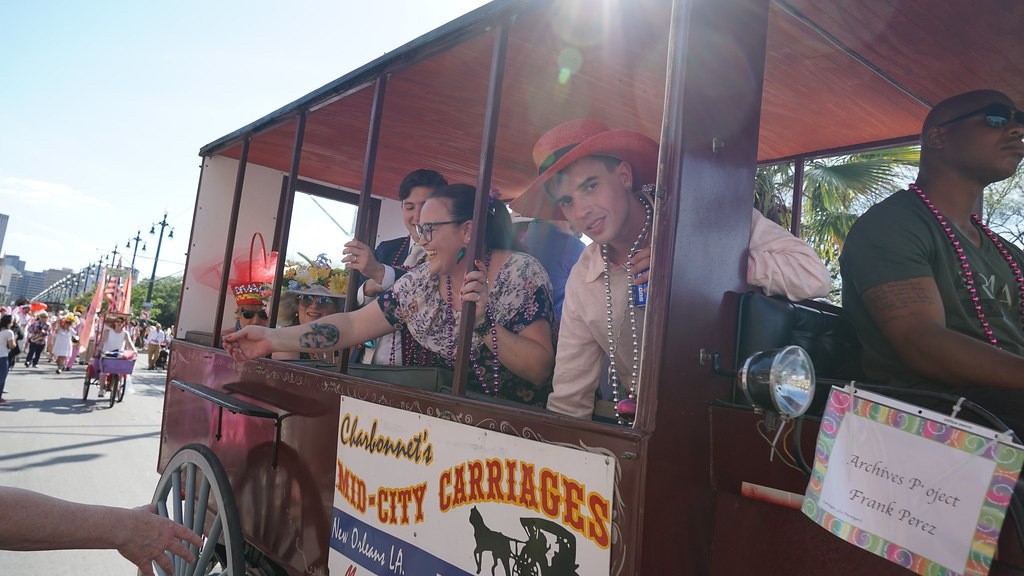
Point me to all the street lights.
[27,260,96,306]
[124,230,147,276]
[143,208,177,311]
[103,244,123,284]
[90,256,107,287]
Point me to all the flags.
[79,261,131,353]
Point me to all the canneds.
[630,250,647,308]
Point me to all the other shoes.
[56,369,60,374]
[149,366,154,370]
[25,362,29,367]
[0,399,6,404]
[32,364,39,368]
[98,391,103,397]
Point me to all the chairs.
[711,289,1023,537]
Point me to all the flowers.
[253,252,348,306]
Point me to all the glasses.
[241,310,269,320]
[415,215,472,242]
[116,321,124,324]
[937,103,1024,129]
[297,294,339,308]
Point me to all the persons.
[0,484,204,576]
[222,182,560,409]
[516,219,587,326]
[221,281,273,337]
[342,169,447,366]
[509,118,830,421]
[0,304,102,404]
[837,90,1024,444]
[95,316,138,398]
[272,253,348,364]
[128,319,174,370]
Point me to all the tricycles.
[82,310,138,407]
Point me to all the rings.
[486,278,490,284]
[476,296,481,302]
[354,257,357,263]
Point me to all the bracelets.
[473,312,492,336]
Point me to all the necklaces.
[389,233,436,368]
[443,247,498,398]
[603,196,652,426]
[909,183,1024,349]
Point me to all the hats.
[509,119,660,221]
[267,256,348,327]
[228,232,274,306]
[0,307,6,312]
